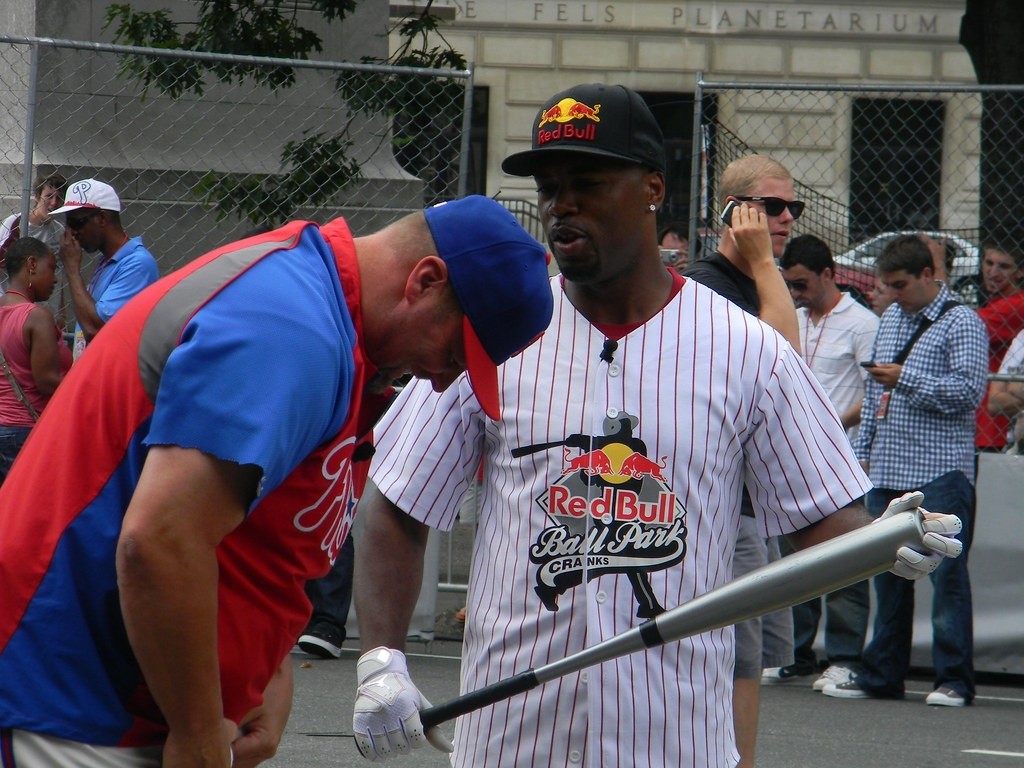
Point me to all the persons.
[0,169,69,333]
[1,194,554,768]
[0,237,73,488]
[297,535,354,658]
[349,83,963,768]
[658,153,1023,707]
[48,177,160,366]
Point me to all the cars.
[831,229,981,306]
[831,258,880,311]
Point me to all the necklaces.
[6,290,31,302]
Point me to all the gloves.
[870,490,964,582]
[351,644,454,761]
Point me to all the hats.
[423,194,555,422]
[46,178,122,214]
[500,82,667,176]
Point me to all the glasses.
[785,280,810,292]
[736,196,806,219]
[63,209,101,231]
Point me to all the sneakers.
[298,627,342,660]
[761,666,820,687]
[812,665,858,691]
[822,679,873,700]
[925,685,967,707]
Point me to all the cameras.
[659,249,680,263]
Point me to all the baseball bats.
[353,508,932,759]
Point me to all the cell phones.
[721,201,737,229]
[860,361,876,368]
